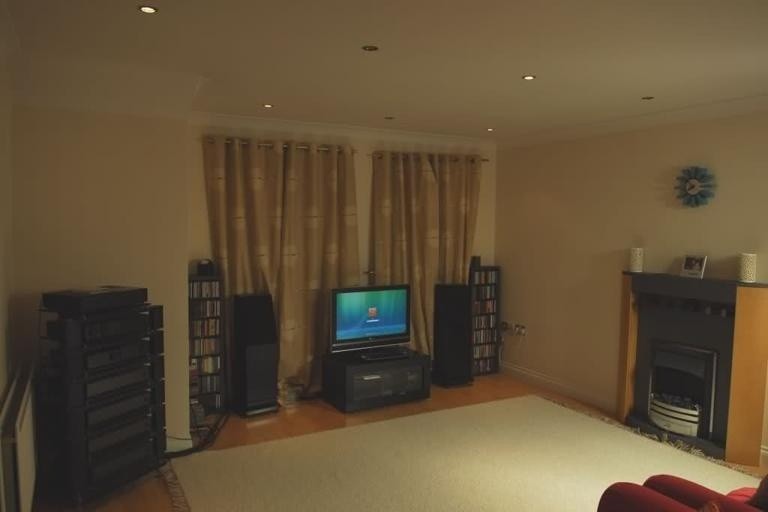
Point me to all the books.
[470,268,497,373]
[189,280,223,410]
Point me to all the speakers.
[434,284,473,386]
[233,293,279,418]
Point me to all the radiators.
[0,360,37,512]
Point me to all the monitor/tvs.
[328,284,413,364]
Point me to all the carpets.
[158,390,764,512]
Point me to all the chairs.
[597,473,768,512]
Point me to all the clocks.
[673,166,718,207]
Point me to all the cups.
[737,252,757,285]
[629,247,643,274]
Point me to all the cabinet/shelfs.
[322,346,431,413]
[36,304,167,511]
[470,266,500,375]
[189,274,227,451]
